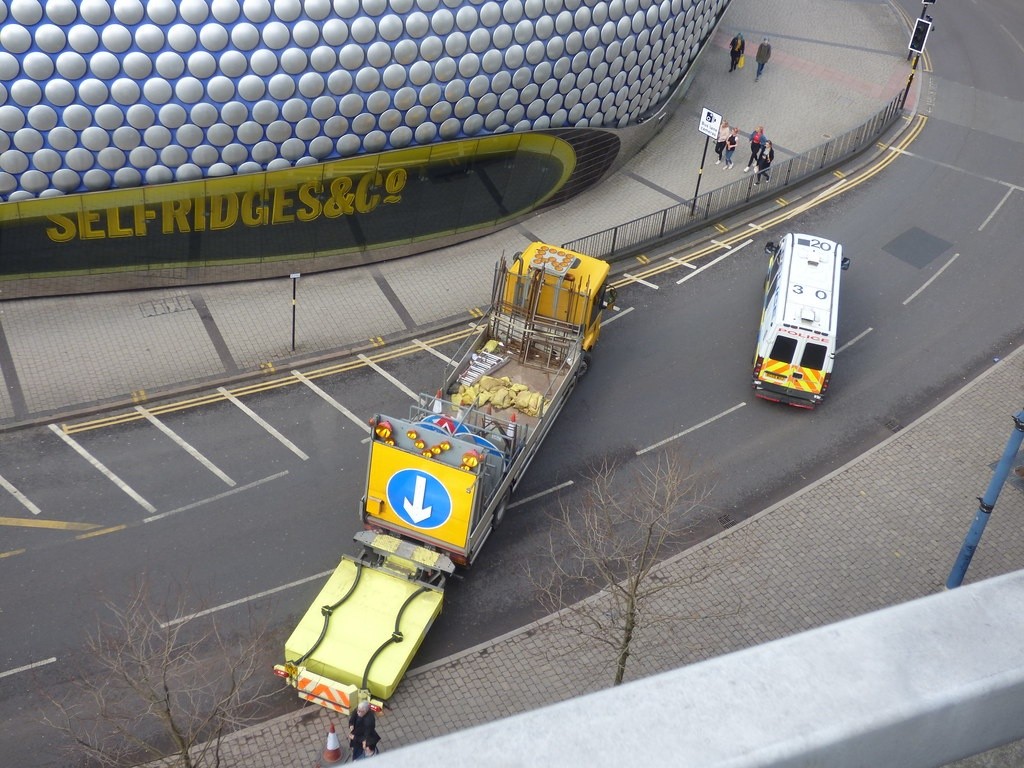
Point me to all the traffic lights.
[908,18,931,54]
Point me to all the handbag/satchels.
[738,55,744,68]
[754,165,759,173]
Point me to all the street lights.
[290,272,300,350]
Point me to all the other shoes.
[744,166,750,172]
[715,160,721,164]
[766,176,771,182]
[734,64,736,70]
[729,163,734,169]
[759,71,761,75]
[723,165,728,169]
[753,161,757,167]
[754,182,760,185]
[729,69,733,72]
[755,78,758,81]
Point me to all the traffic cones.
[322,721,343,763]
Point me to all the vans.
[751,233,849,411]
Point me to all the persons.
[754,37,771,82]
[743,125,775,185]
[714,120,739,170]
[347,701,381,763]
[728,32,745,72]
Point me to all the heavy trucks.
[353,240,619,584]
